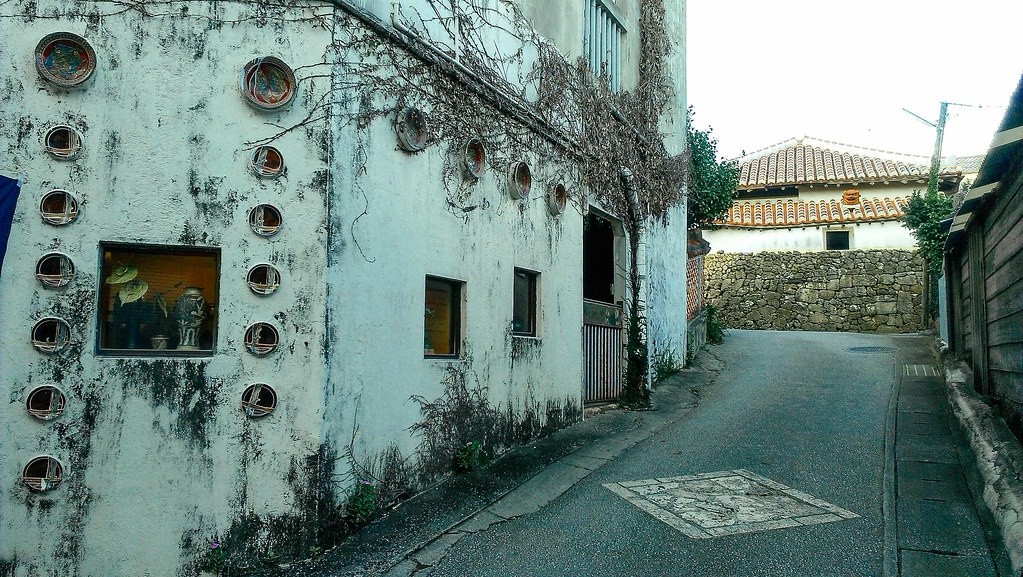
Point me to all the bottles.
[173,287,208,350]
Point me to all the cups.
[150,337,170,349]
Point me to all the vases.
[150,334,170,349]
[172,284,208,349]
